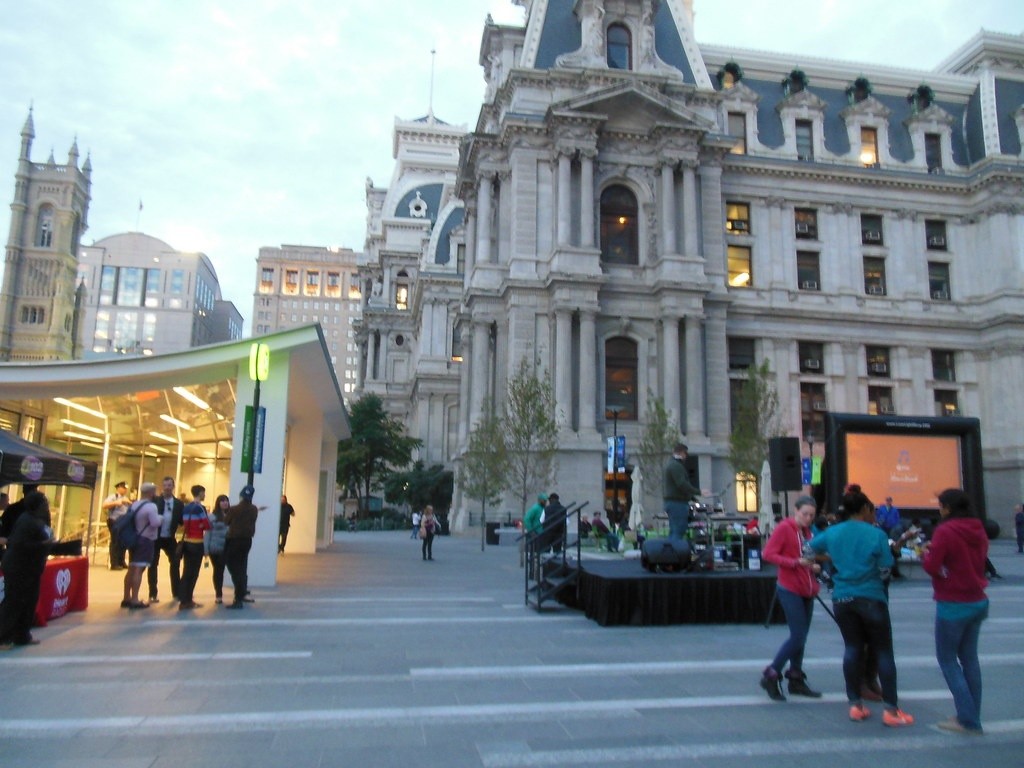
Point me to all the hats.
[115,481,129,490]
[537,494,548,501]
[548,493,559,500]
[22,491,45,511]
[240,484,255,502]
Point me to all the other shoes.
[25,636,40,645]
[110,593,255,614]
[938,716,984,738]
[991,573,1003,579]
[860,684,883,705]
[0,641,14,651]
[869,678,886,697]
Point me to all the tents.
[0,426,98,558]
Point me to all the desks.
[0,558,88,625]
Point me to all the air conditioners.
[929,236,945,246]
[734,221,744,229]
[880,405,896,415]
[813,401,827,411]
[805,358,819,369]
[872,362,886,372]
[802,281,817,289]
[796,223,808,234]
[935,290,949,300]
[866,231,880,241]
[868,285,883,295]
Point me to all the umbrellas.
[628,464,643,553]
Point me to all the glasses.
[166,501,171,512]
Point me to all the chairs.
[589,524,612,551]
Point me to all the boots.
[784,670,822,697]
[759,667,787,702]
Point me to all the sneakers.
[849,705,871,723]
[884,708,915,728]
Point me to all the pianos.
[689,502,724,518]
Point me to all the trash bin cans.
[486,522,500,545]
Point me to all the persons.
[856,629,884,702]
[746,483,1003,584]
[661,443,712,542]
[921,488,990,738]
[524,493,618,579]
[803,484,915,726]
[0,477,258,646]
[409,505,441,560]
[278,495,295,554]
[760,496,822,702]
[1014,504,1024,553]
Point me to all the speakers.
[769,437,803,492]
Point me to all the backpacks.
[116,500,152,548]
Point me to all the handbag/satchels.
[175,540,183,561]
[418,516,427,540]
